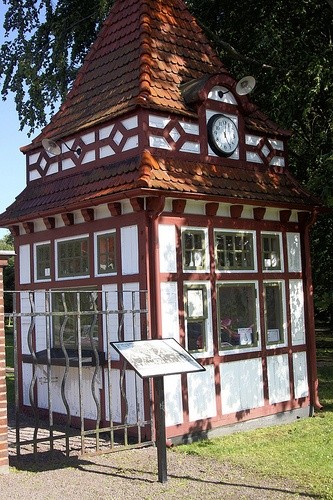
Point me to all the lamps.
[218,76,256,99]
[42,137,81,156]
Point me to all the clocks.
[207,113,239,157]
